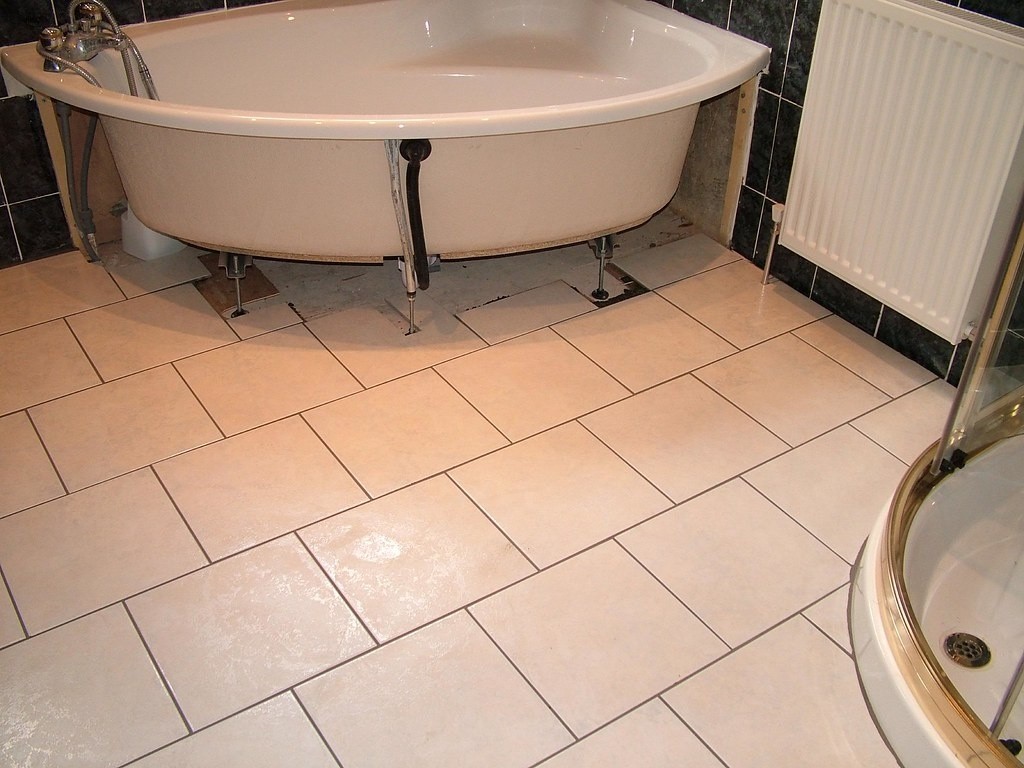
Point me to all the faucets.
[64,1,128,57]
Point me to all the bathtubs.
[0,0,771,264]
